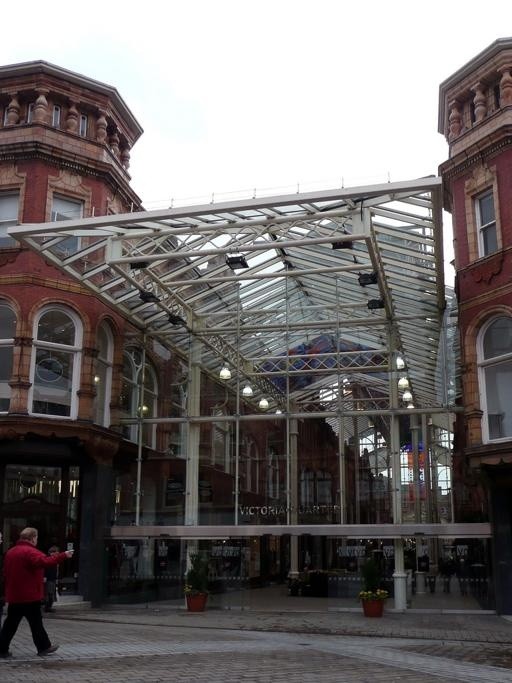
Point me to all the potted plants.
[184,595,208,612]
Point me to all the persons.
[1,527,75,658]
[44,547,59,613]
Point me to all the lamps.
[220,363,282,415]
[130,241,385,325]
[396,357,415,408]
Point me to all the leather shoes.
[1,652,13,659]
[38,644,59,655]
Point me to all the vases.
[362,598,384,617]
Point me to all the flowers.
[184,552,209,596]
[359,550,389,600]
